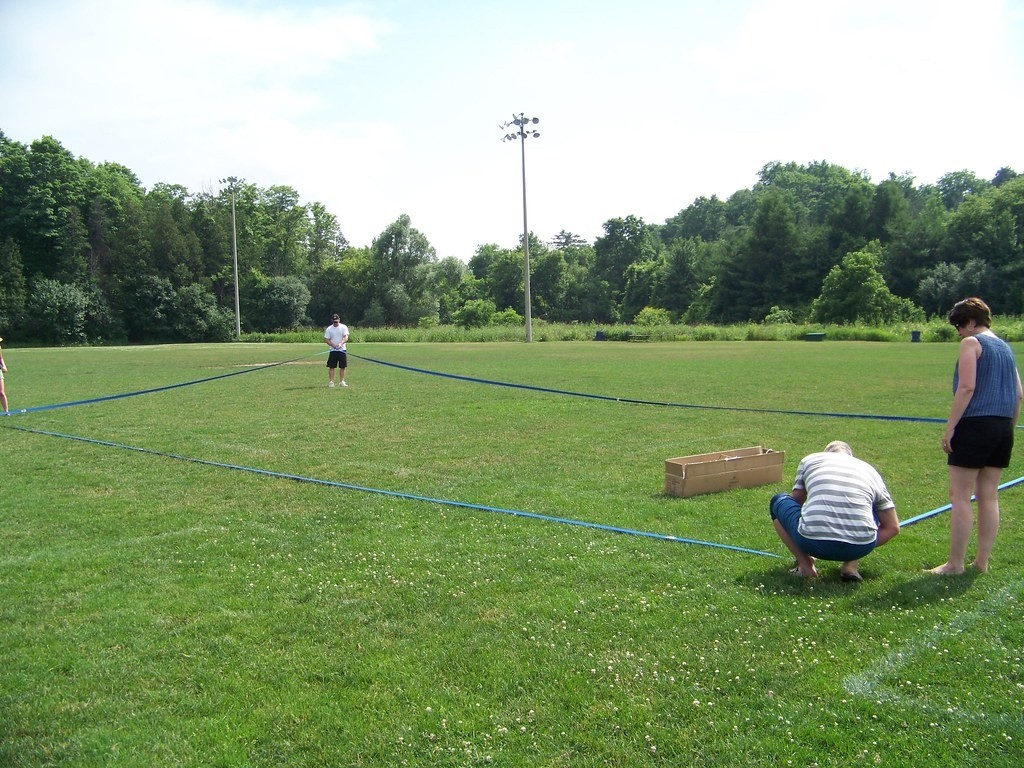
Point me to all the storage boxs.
[665,445,785,497]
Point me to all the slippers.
[841,571,863,583]
[788,565,803,577]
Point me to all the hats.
[331,314,339,321]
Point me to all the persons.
[325,314,349,387]
[922,298,1023,575]
[769,441,900,582]
[0,338,10,415]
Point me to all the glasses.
[956,325,961,331]
[333,321,338,323]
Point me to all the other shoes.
[6,412,10,416]
[339,381,348,387]
[329,381,335,387]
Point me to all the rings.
[944,443,946,447]
